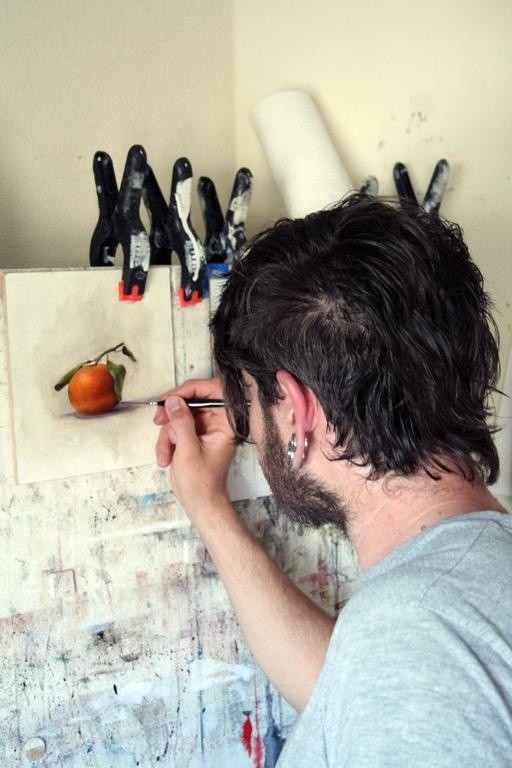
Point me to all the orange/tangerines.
[54,341,136,416]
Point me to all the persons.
[151,176,511,768]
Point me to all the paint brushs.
[116,400,251,408]
